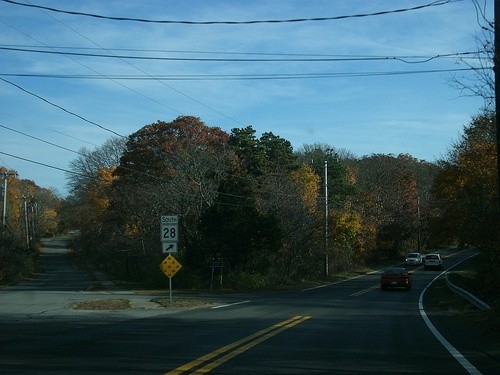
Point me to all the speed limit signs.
[161,223,179,243]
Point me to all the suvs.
[380,266,412,291]
[423,253,443,270]
[404,252,422,265]
